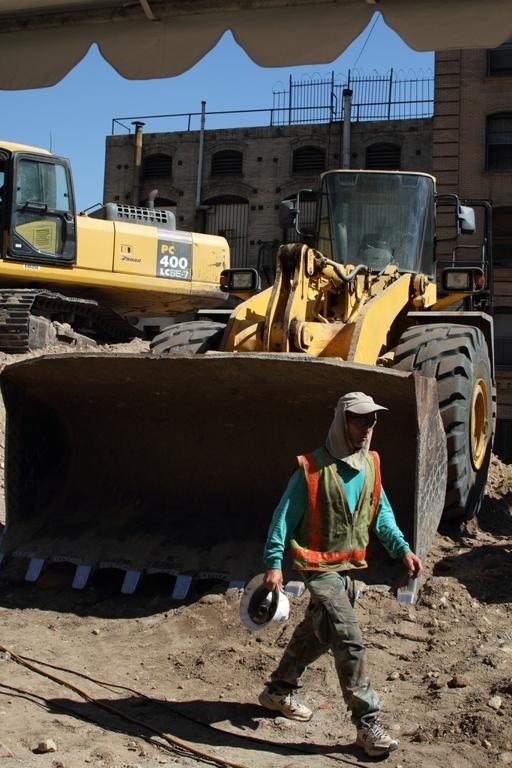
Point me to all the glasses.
[348,414,378,428]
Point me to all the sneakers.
[259,685,313,723]
[356,722,399,757]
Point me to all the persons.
[253,389,424,760]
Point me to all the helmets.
[238,574,290,632]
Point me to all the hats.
[325,391,390,473]
